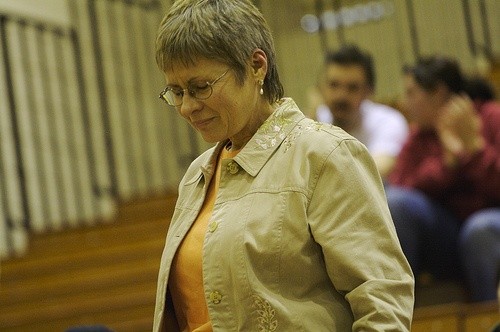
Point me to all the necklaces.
[227,145,232,151]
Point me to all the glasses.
[159,67,231,106]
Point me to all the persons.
[153,0,415,332]
[385,53,500,309]
[305,40,409,186]
